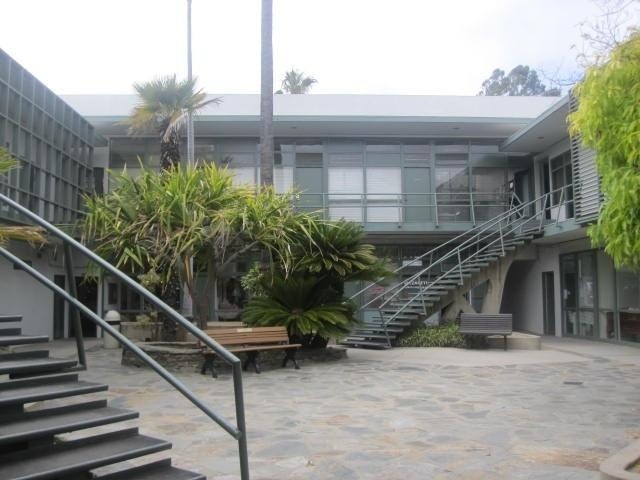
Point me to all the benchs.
[438,213,457,221]
[455,312,512,352]
[199,326,302,379]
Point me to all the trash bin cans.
[104,310,121,349]
